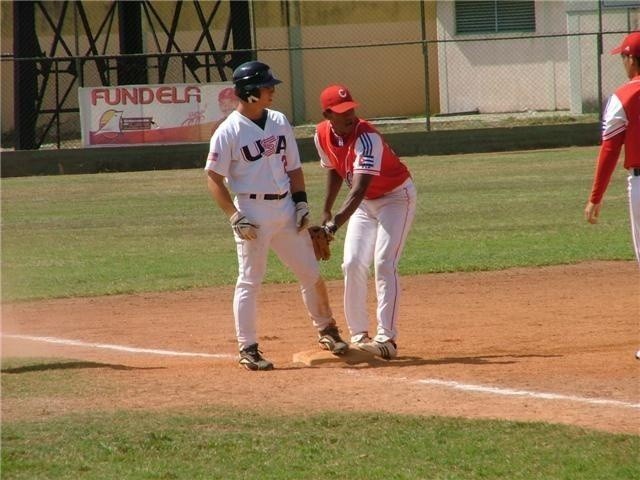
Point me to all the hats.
[607,31,640,58]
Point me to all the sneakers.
[238,342,273,372]
[317,324,348,354]
[357,339,398,360]
[357,336,372,343]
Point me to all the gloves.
[228,211,261,242]
[295,201,311,232]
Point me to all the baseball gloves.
[307,224,333,261]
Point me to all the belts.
[250,190,289,200]
[633,169,640,177]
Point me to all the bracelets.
[292,192,307,204]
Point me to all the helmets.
[319,84,360,114]
[232,61,282,104]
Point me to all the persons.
[204,61,349,370]
[584,32,639,359]
[210,87,239,135]
[307,85,417,358]
